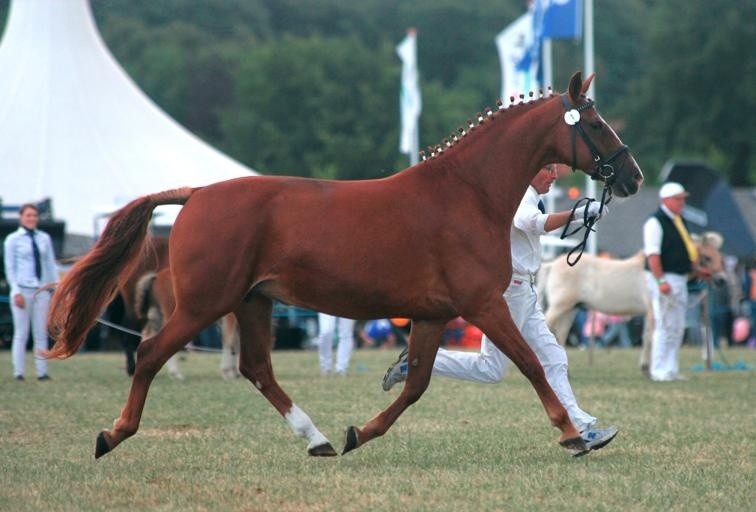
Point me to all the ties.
[29,231,41,281]
[537,200,546,216]
[672,214,698,262]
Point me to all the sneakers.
[580,426,619,454]
[381,347,409,391]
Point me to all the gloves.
[572,200,610,221]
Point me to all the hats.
[659,182,689,200]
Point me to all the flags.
[394,25,425,157]
[493,0,589,111]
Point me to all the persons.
[0,201,62,382]
[313,311,361,378]
[359,233,756,348]
[380,161,619,461]
[639,179,713,385]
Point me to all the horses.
[531,235,727,377]
[36,72,644,460]
[61,235,245,384]
